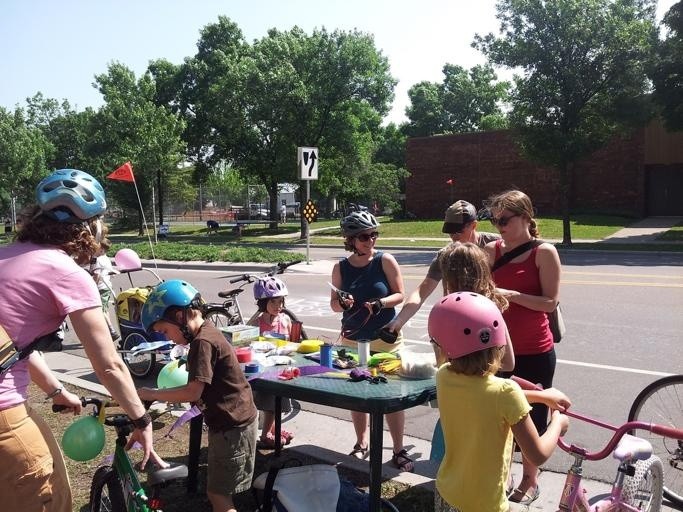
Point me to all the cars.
[328,202,368,219]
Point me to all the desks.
[181,338,445,512]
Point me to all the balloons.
[155,358,189,390]
[114,248,142,271]
[60,414,105,462]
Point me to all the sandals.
[392,449,415,474]
[349,443,369,461]
[505,478,540,507]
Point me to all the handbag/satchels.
[545,300,566,344]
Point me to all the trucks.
[228,202,269,220]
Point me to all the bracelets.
[43,382,64,401]
[129,411,153,430]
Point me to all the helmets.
[427,291,507,360]
[35,169,107,220]
[140,279,202,332]
[253,277,288,300]
[340,210,380,237]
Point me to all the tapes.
[244,364,259,373]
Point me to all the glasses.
[354,231,378,242]
[489,213,521,225]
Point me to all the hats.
[441,199,478,233]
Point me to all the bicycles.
[50,396,188,512]
[507,371,683,512]
[627,375,683,509]
[199,259,308,341]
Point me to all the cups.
[319,343,332,369]
[234,346,251,363]
[357,339,370,367]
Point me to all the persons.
[426,289,572,510]
[427,239,517,476]
[381,199,502,339]
[480,188,562,510]
[328,209,416,476]
[0,166,172,511]
[89,237,120,342]
[205,219,219,236]
[243,276,295,450]
[133,276,260,511]
[279,203,286,225]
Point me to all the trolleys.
[83,264,203,377]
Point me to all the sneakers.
[111,331,121,342]
[260,433,287,448]
[273,427,294,440]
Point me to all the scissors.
[327,281,354,308]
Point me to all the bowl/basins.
[397,343,438,379]
